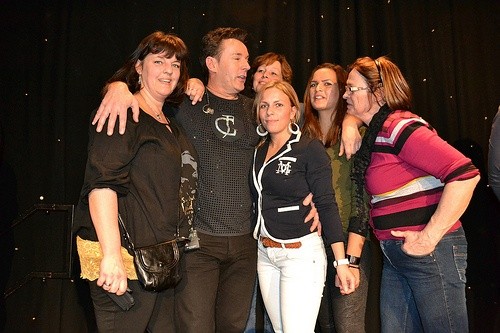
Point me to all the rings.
[103,282,112,286]
[193,89,198,91]
[111,288,118,290]
[188,88,193,91]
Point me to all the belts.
[260,237,302,248]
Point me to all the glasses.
[345,85,368,92]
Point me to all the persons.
[343,56,482,333]
[75,25,383,333]
[487,105,500,199]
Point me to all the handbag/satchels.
[133,239,183,291]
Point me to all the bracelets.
[345,253,361,269]
[333,259,349,268]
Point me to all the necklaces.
[139,89,163,121]
